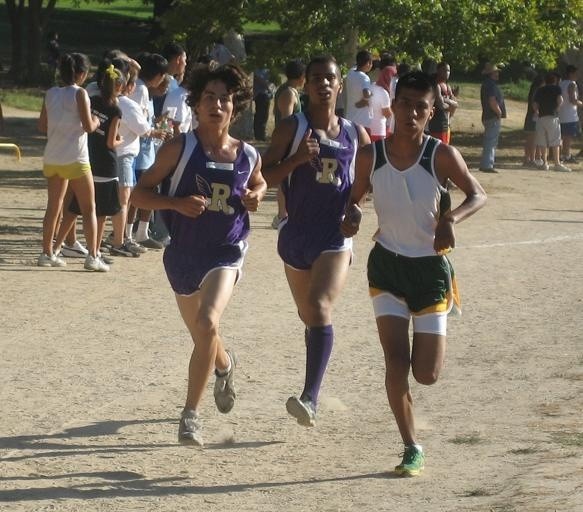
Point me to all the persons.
[479,62,506,173]
[131,63,267,450]
[38,53,112,270]
[521,64,583,171]
[54,65,124,263]
[337,70,487,479]
[86,42,217,257]
[261,56,375,427]
[273,50,458,236]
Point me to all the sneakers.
[480,154,582,173]
[37,234,172,271]
[272,215,287,229]
[394,442,426,477]
[286,396,317,427]
[214,347,238,413]
[178,410,203,449]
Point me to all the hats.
[481,62,502,75]
